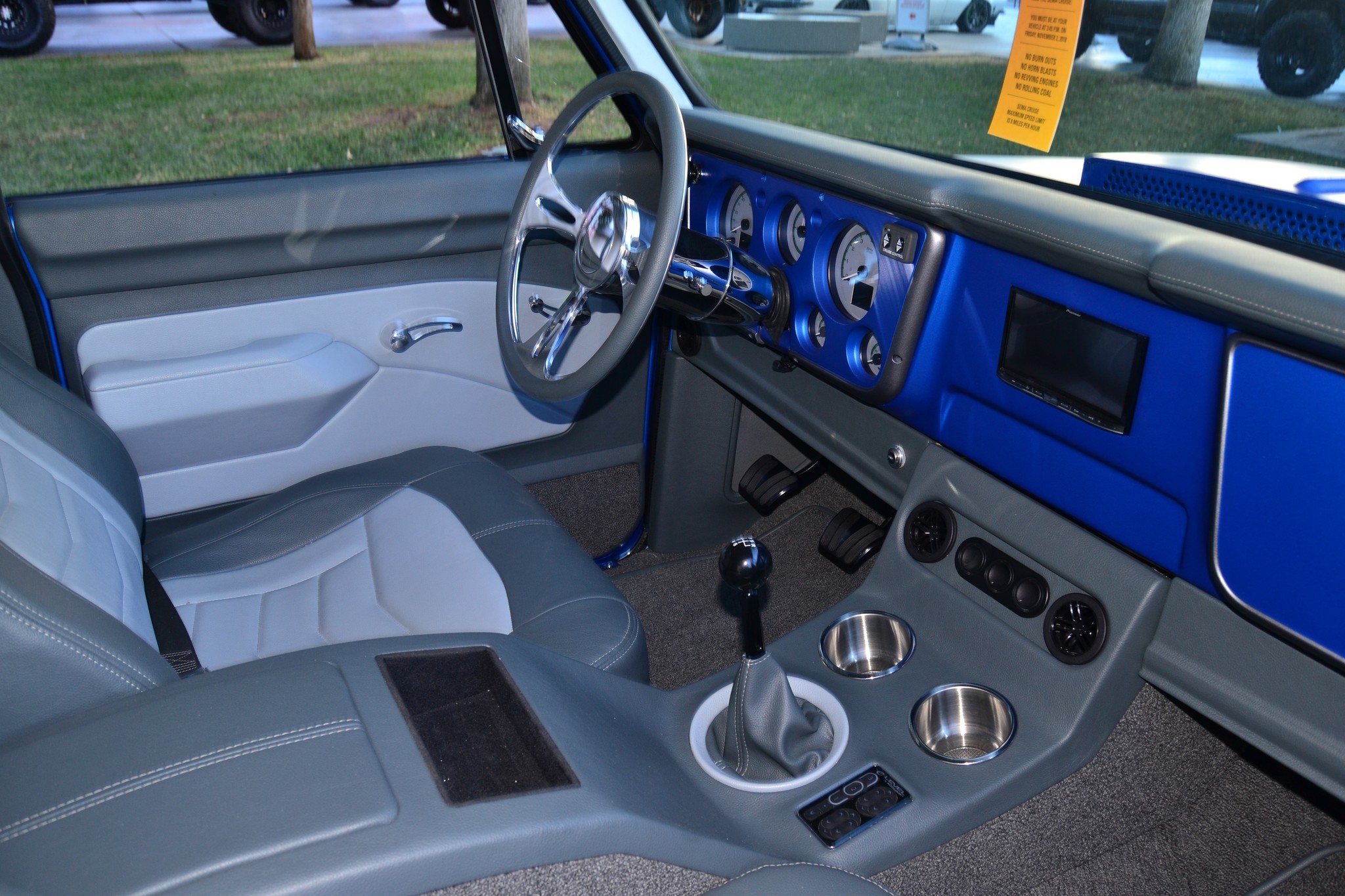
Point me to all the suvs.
[1075,2,1344,99]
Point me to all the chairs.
[0,351,650,744]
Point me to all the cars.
[796,1,1007,33]
